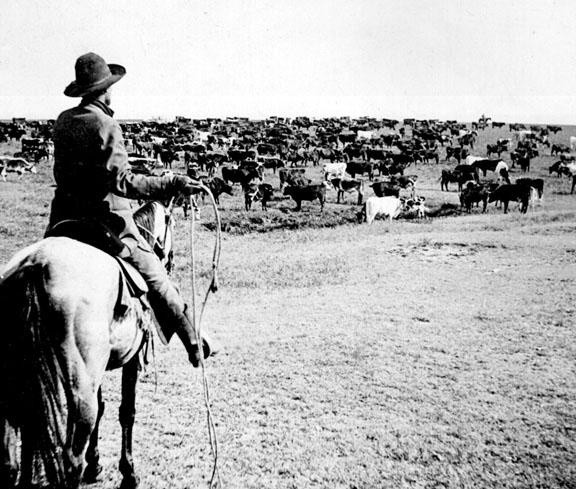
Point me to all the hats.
[64,52,126,97]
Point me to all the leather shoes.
[189,339,209,367]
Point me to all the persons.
[44,53,219,368]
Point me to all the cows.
[0,118,56,183]
[119,117,576,226]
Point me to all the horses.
[0,196,177,489]
